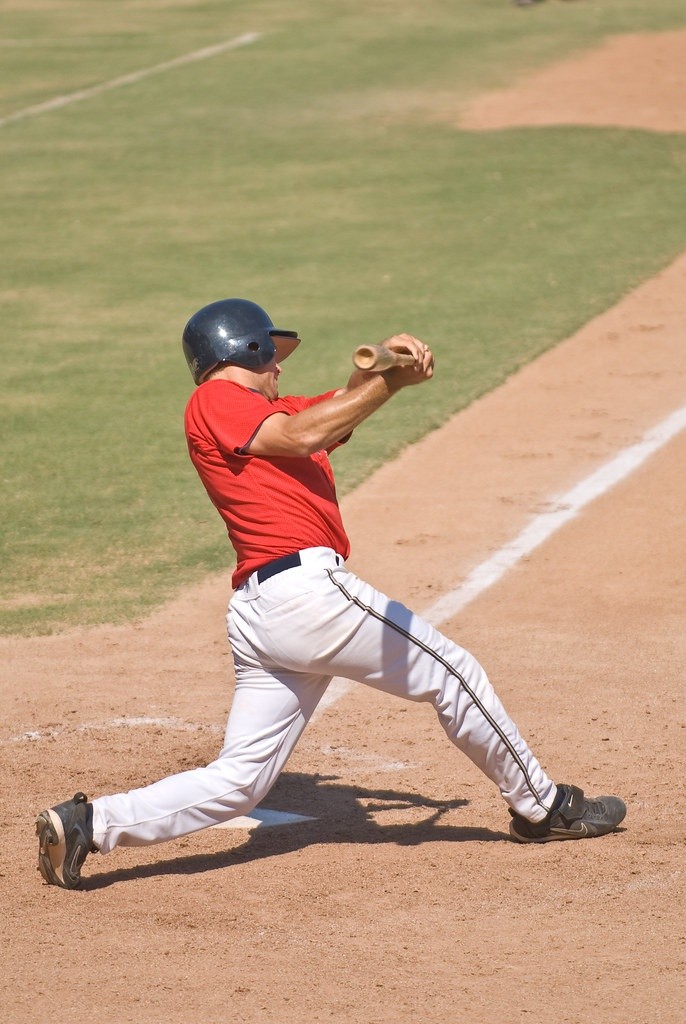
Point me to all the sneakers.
[36,791,97,889]
[508,784,627,844]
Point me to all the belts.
[260,553,342,579]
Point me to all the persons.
[34,299,627,888]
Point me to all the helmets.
[181,297,299,388]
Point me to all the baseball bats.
[352,344,415,371]
